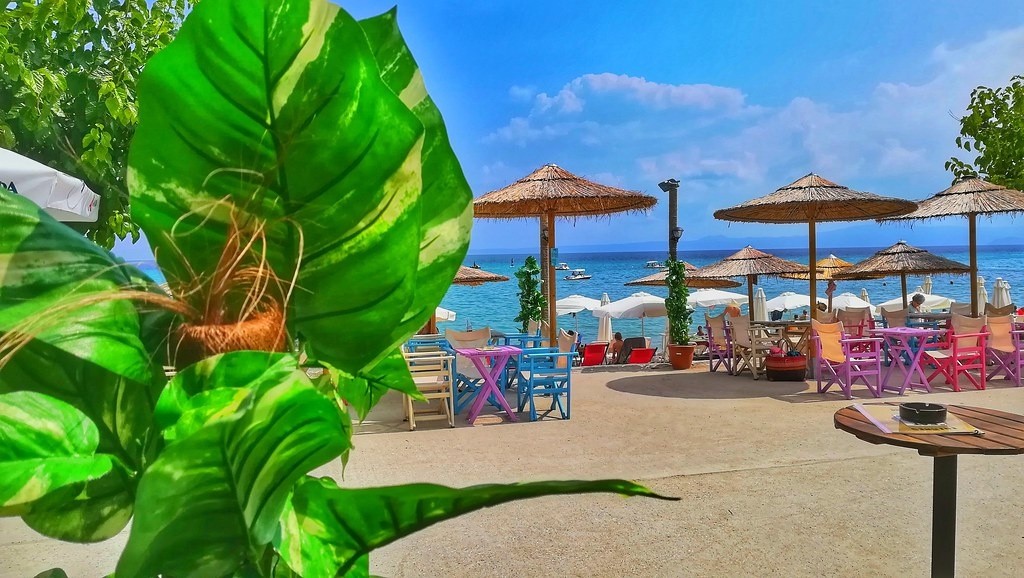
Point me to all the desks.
[834,401,1024,578]
[865,327,947,395]
[750,320,810,375]
[908,312,951,322]
[455,346,522,424]
[490,329,503,345]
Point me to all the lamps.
[671,227,684,242]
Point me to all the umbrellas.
[916,277,931,295]
[453,265,510,285]
[593,291,694,336]
[977,276,1011,316]
[713,172,916,324]
[876,177,1024,317]
[0,146,101,222]
[686,289,749,313]
[861,290,869,302]
[598,293,612,341]
[876,292,956,314]
[436,306,456,322]
[556,294,601,332]
[474,163,657,349]
[767,292,880,316]
[625,240,970,310]
[754,287,769,335]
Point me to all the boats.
[646,261,666,268]
[564,268,591,280]
[470,261,481,269]
[555,262,570,270]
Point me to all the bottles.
[488,339,492,350]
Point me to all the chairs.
[575,337,658,366]
[812,302,1024,399]
[403,318,580,432]
[704,312,786,380]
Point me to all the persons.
[697,326,709,347]
[909,293,925,322]
[723,303,741,317]
[800,310,807,319]
[613,332,624,358]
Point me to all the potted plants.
[665,258,697,370]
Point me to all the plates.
[476,347,501,351]
